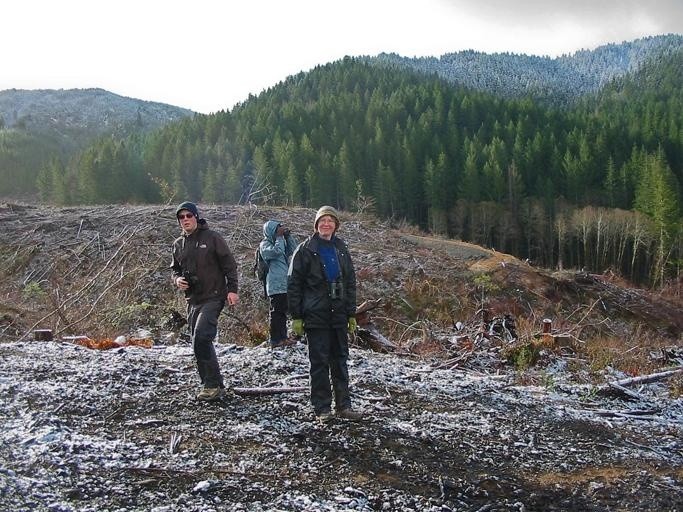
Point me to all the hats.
[176,201,199,226]
[314,206,340,229]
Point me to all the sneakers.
[272,338,297,349]
[197,384,225,401]
[316,407,363,422]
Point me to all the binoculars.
[183,271,200,287]
[283,228,290,235]
[331,279,344,300]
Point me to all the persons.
[169,200,241,402]
[287,204,366,423]
[260,220,299,348]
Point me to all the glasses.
[178,212,194,220]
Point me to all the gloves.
[292,320,305,335]
[277,226,290,236]
[348,318,357,334]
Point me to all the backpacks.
[252,238,275,281]
